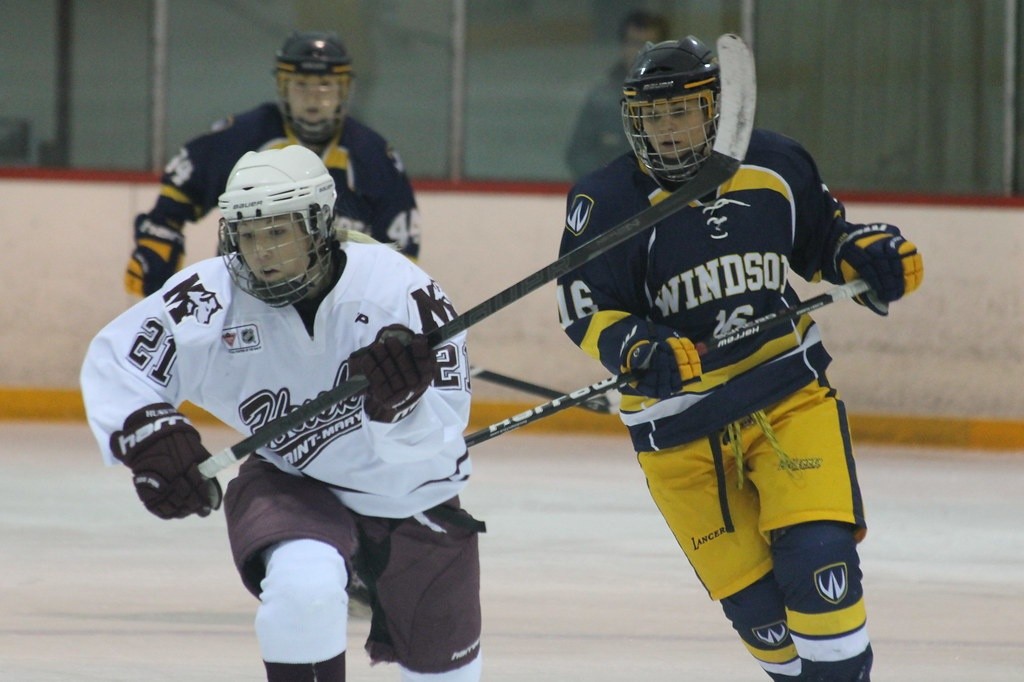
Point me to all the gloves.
[124,213,186,298]
[597,313,702,401]
[824,218,923,316]
[348,323,439,424]
[109,402,214,519]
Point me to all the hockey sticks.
[462,277,872,448]
[196,32,758,484]
[469,363,623,415]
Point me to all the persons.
[81,145,486,682]
[557,37,923,682]
[566,9,675,175]
[124,32,423,442]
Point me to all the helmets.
[620,34,721,182]
[270,31,358,141]
[217,144,337,308]
